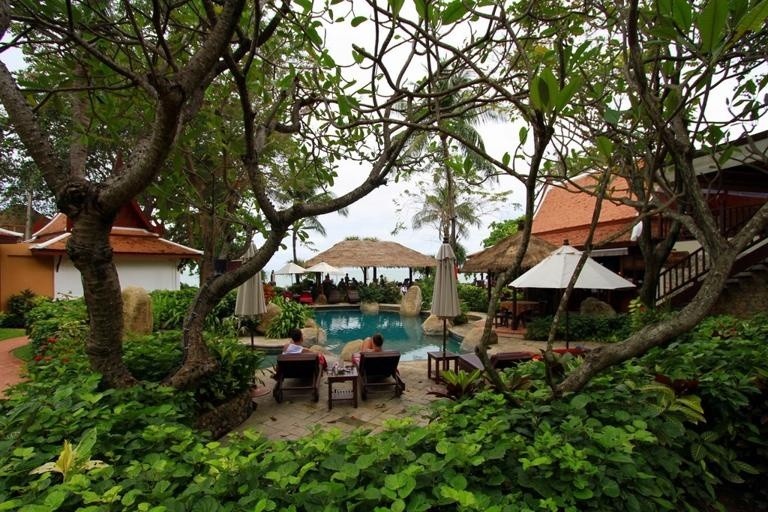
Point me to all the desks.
[327,364,359,408]
[428,348,456,379]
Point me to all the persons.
[323,276,332,284]
[398,282,403,294]
[280,328,318,356]
[352,278,357,284]
[380,275,386,286]
[360,333,385,353]
[345,273,350,283]
[403,278,409,285]
[338,278,344,286]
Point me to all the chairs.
[354,350,405,402]
[275,351,324,402]
[493,307,534,330]
[458,346,594,384]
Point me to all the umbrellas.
[236,241,268,388]
[431,231,462,371]
[507,238,638,350]
[274,262,306,286]
[306,260,339,296]
[458,218,561,331]
